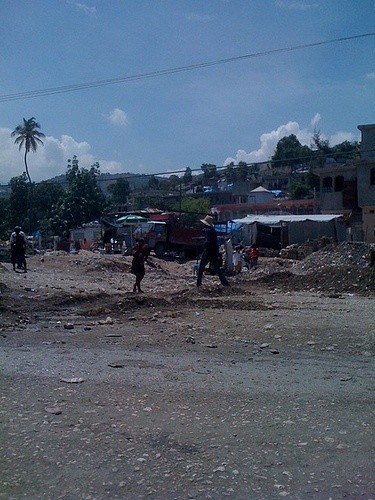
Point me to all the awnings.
[231,214,344,225]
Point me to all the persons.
[9,215,258,293]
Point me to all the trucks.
[133,210,217,260]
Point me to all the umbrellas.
[116,214,147,246]
